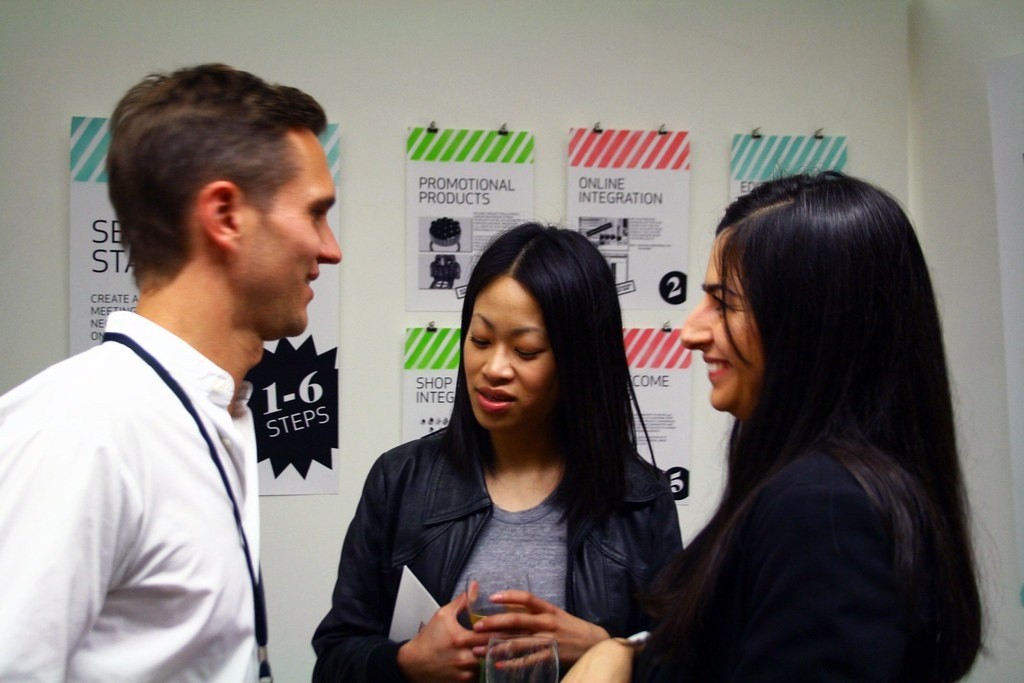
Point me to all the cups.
[465,568,559,683]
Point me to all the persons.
[310,220,682,683]
[0,63,344,683]
[557,168,983,683]
